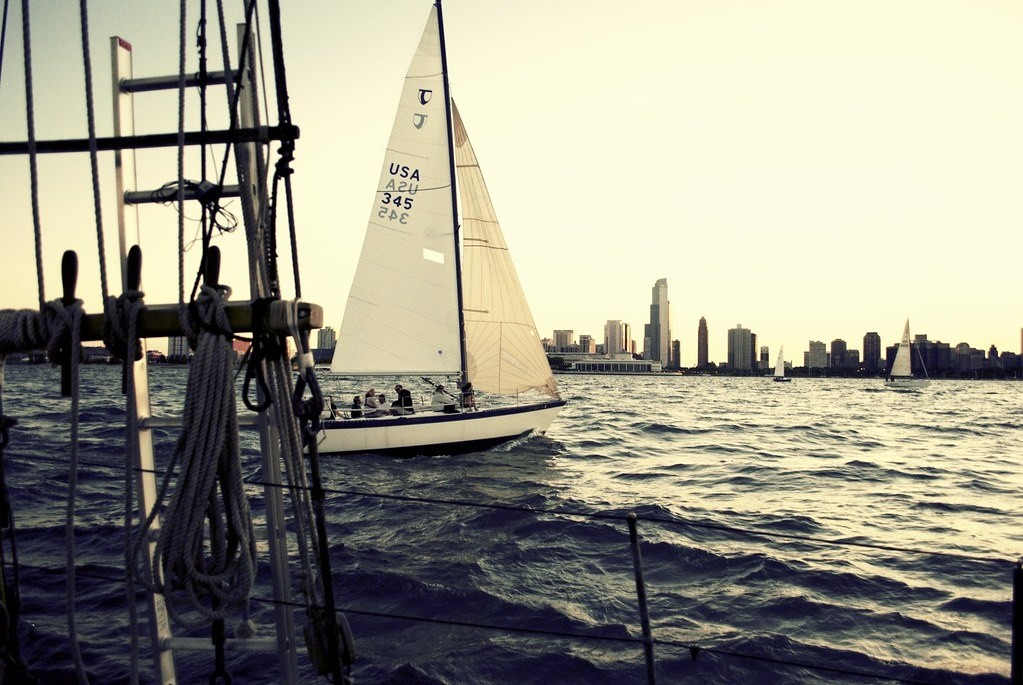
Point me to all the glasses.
[396,388,400,391]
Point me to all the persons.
[391,385,415,416]
[431,385,455,411]
[324,395,344,420]
[364,388,390,418]
[351,396,362,418]
[461,383,478,412]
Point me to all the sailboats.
[305,0,569,458]
[884,316,932,388]
[773,344,791,381]
